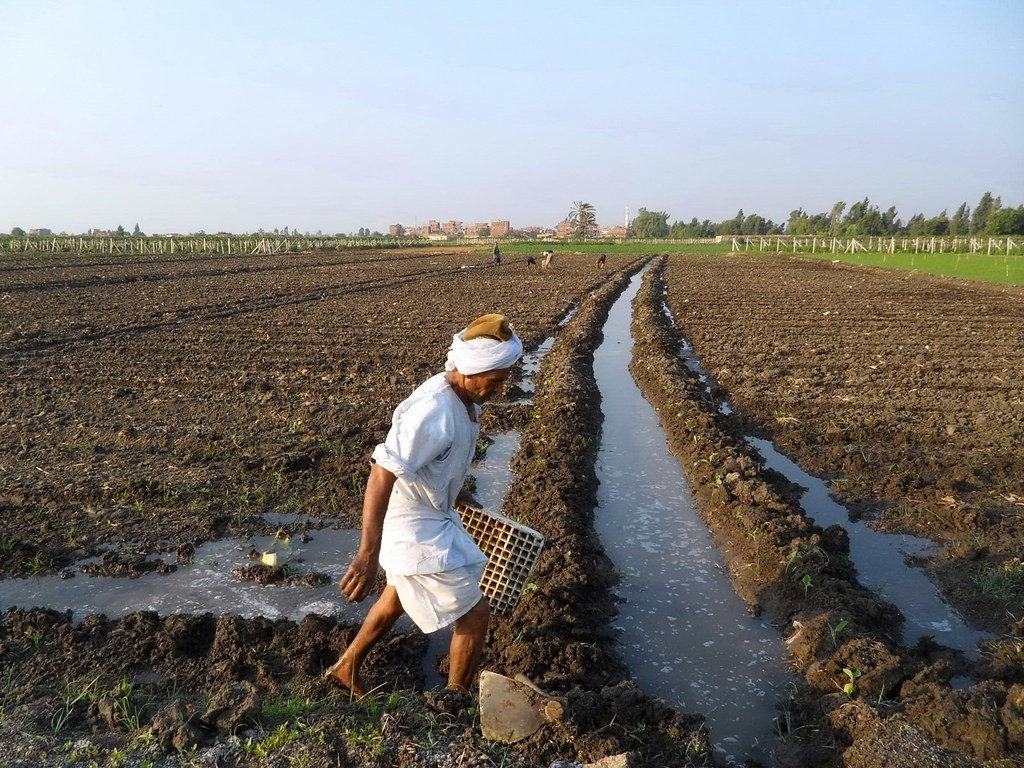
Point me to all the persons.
[527,256,536,270]
[595,254,606,270]
[493,242,502,267]
[325,314,523,699]
[543,250,554,269]
